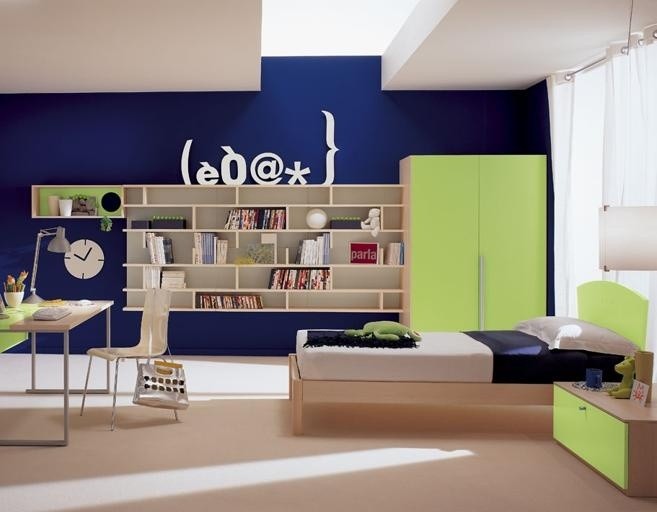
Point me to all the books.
[378,242,404,263]
[142,209,331,308]
[33,307,71,321]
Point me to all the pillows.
[512,315,640,357]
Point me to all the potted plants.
[59,194,73,216]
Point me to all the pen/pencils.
[3,280,26,292]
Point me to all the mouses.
[77,299,91,304]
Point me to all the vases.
[4,292,25,307]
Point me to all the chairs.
[80,287,178,432]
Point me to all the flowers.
[3,270,29,292]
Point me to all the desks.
[0,300,114,447]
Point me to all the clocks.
[63,238,105,280]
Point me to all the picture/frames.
[348,241,379,264]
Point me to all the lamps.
[22,226,71,304]
[598,205,657,273]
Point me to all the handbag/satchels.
[133,344,189,410]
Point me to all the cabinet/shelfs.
[121,184,411,329]
[399,154,548,331]
[31,184,127,220]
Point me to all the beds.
[287,280,649,439]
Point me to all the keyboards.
[34,306,71,321]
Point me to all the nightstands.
[552,381,657,496]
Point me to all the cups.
[58,199,72,216]
[586,368,603,389]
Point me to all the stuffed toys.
[360,209,381,238]
[343,321,422,341]
[609,357,634,398]
